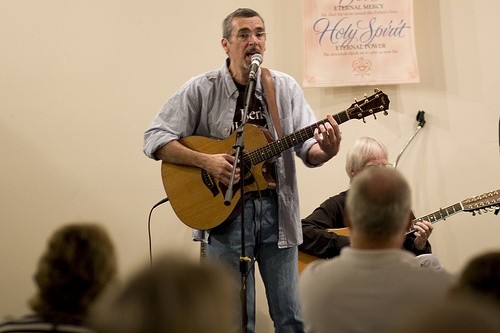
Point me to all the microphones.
[249,53,263,81]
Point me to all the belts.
[243,189,278,198]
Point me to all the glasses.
[223,31,267,43]
[365,163,394,167]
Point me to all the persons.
[297,136,500,333]
[0,225,114,333]
[142,8,340,333]
[114,250,240,333]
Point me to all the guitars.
[298,188,500,274]
[161,88,390,230]
